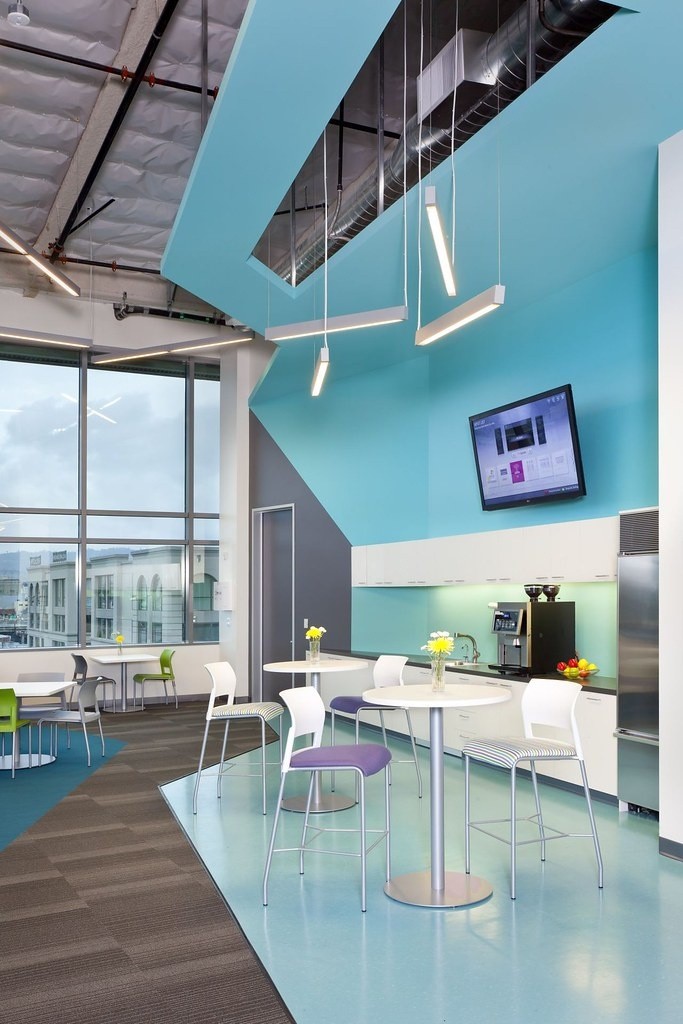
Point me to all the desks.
[0,680,77,770]
[263,658,371,813]
[362,685,511,908]
[91,654,159,712]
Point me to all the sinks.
[444,661,479,667]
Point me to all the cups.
[524,584,544,601]
[543,585,561,601]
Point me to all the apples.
[557,659,589,677]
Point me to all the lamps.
[0,0,504,397]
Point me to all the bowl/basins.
[556,668,601,680]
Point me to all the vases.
[431,662,446,693]
[309,642,321,663]
[118,643,124,655]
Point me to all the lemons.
[563,659,597,676]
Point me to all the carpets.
[0,725,128,858]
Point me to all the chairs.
[0,647,178,779]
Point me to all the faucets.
[453,632,480,664]
[461,644,469,663]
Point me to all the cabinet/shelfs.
[305,651,618,805]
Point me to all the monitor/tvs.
[468,383,586,510]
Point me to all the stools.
[192,655,604,912]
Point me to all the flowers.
[305,626,326,659]
[421,631,455,690]
[111,632,125,653]
